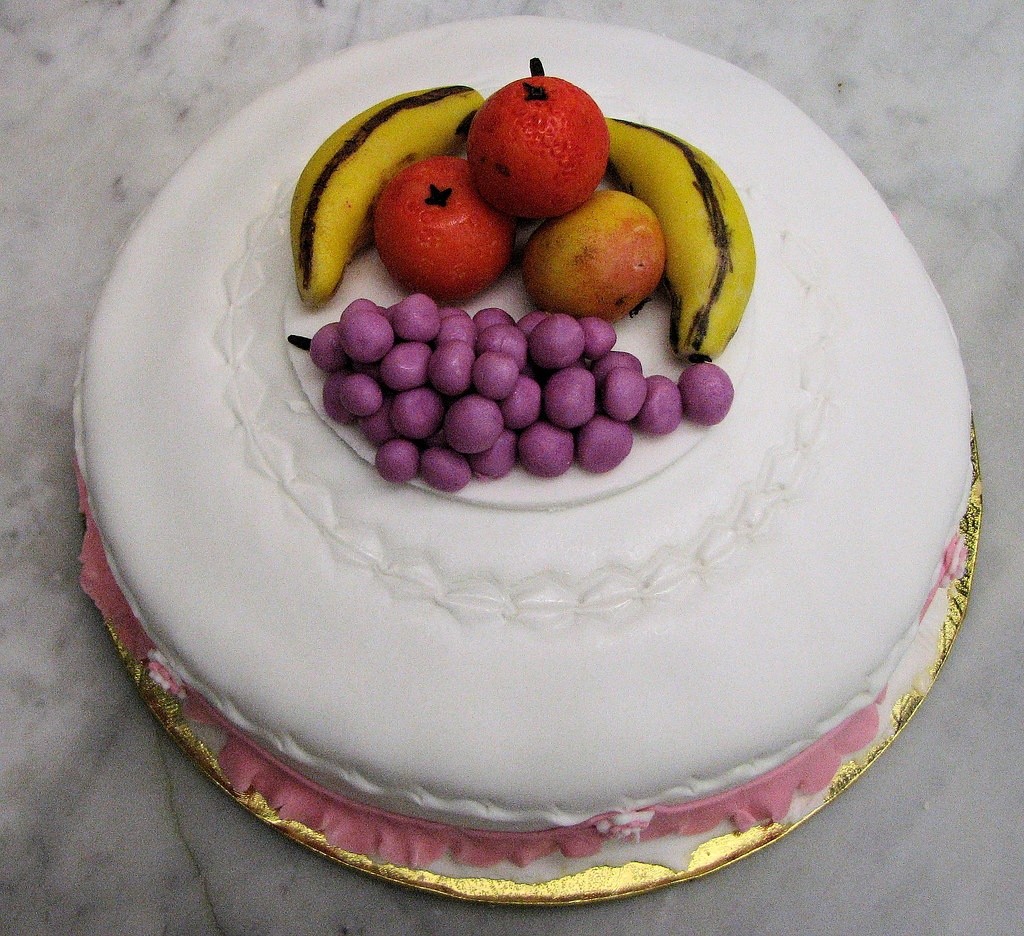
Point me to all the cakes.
[72,11,983,909]
